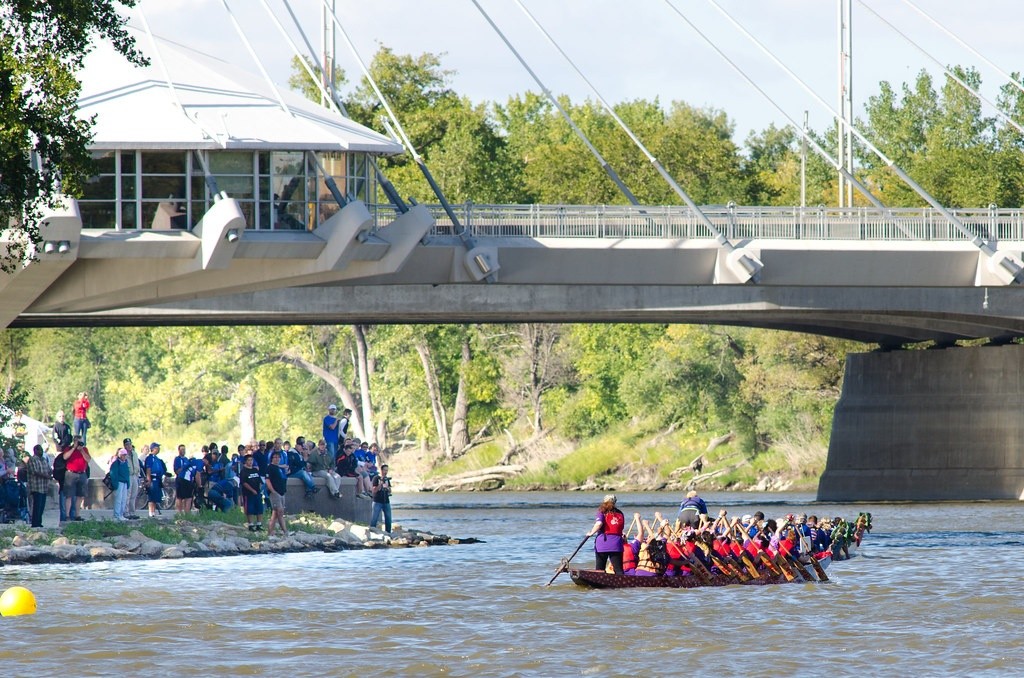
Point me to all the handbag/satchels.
[102,473,115,490]
[84,419,92,428]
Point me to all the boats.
[815,510,873,561]
[561,551,834,583]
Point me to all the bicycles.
[136,472,178,510]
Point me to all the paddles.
[620,509,849,585]
[541,530,591,588]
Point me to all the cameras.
[78,441,84,446]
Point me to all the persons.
[0,392,392,536]
[678,491,708,529]
[587,495,624,575]
[611,510,829,576]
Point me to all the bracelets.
[718,516,721,519]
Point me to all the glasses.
[259,444,265,446]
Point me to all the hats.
[604,494,617,503]
[329,405,336,410]
[118,449,128,458]
[150,443,160,450]
[228,476,241,488]
[123,438,133,444]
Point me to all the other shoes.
[335,492,342,498]
[126,515,139,520]
[31,525,43,527]
[313,488,321,493]
[76,516,86,521]
[304,494,314,500]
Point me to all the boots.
[257,522,265,531]
[249,523,257,531]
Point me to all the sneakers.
[356,494,363,500]
[268,535,281,541]
[116,517,125,522]
[284,531,295,537]
[360,491,371,500]
